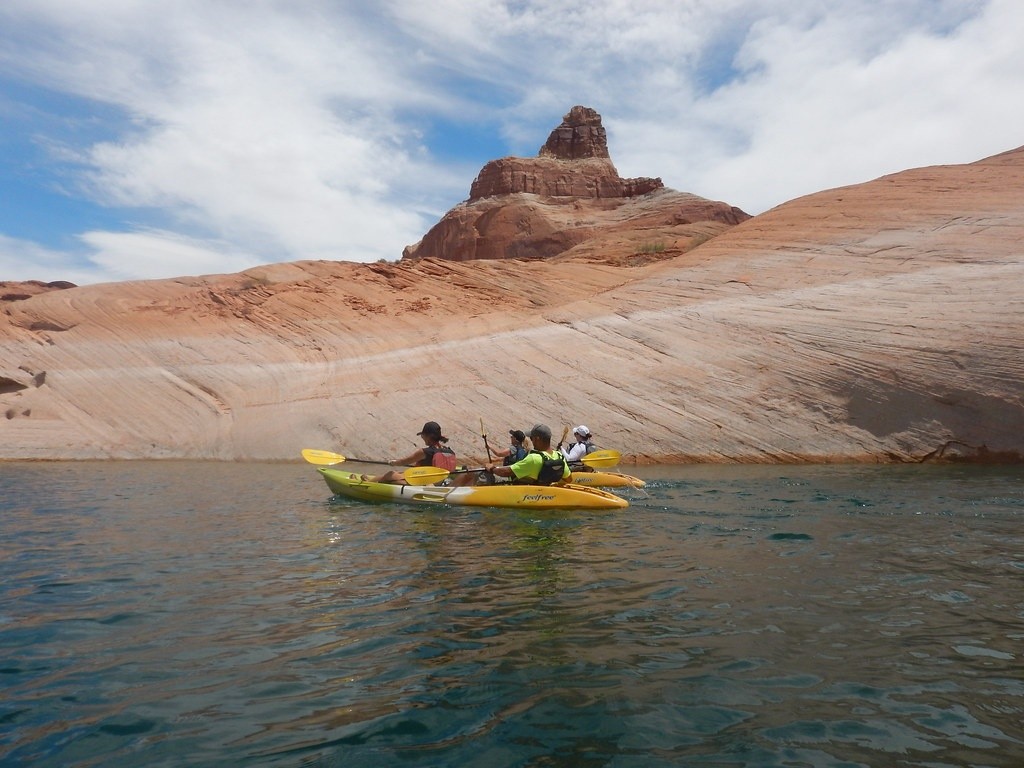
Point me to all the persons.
[557,425,598,473]
[448,423,573,488]
[350,421,456,486]
[485,430,531,466]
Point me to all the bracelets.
[490,466,496,474]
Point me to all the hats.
[417,422,444,438]
[525,423,552,439]
[573,425,590,437]
[509,429,525,443]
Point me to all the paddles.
[480,417,492,463]
[557,426,569,450]
[403,449,621,486]
[301,449,462,470]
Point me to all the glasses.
[511,435,516,439]
[529,437,538,441]
[574,433,578,437]
[420,434,429,438]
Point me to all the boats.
[450,465,648,489]
[316,467,629,509]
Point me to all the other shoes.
[350,474,356,479]
[361,474,369,481]
